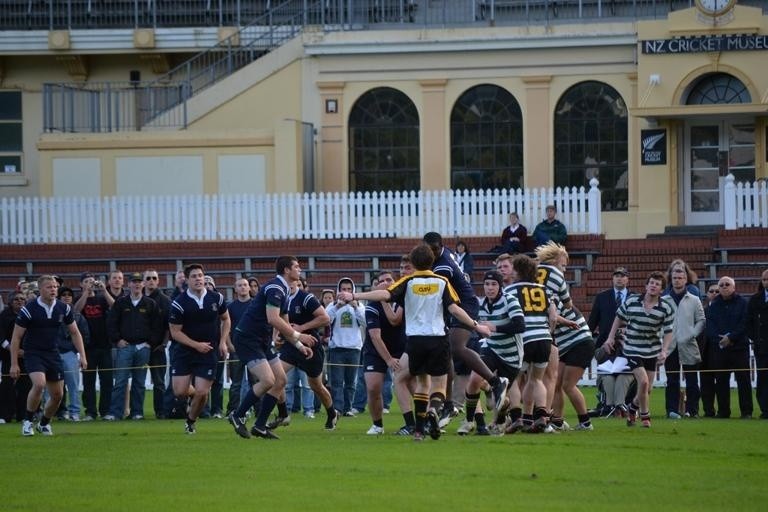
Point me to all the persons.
[227,278,257,420]
[493,213,527,265]
[140,268,172,419]
[10,275,89,438]
[545,295,596,432]
[530,205,568,251]
[452,239,473,288]
[263,280,338,430]
[665,260,701,301]
[297,277,306,292]
[73,272,115,421]
[362,271,403,435]
[227,256,318,441]
[169,270,188,300]
[247,275,261,298]
[0,291,26,423]
[584,266,634,416]
[54,277,65,296]
[15,278,28,295]
[203,274,216,292]
[336,242,491,443]
[28,281,40,301]
[59,287,92,422]
[371,277,379,293]
[318,287,335,308]
[659,269,706,418]
[746,270,768,420]
[169,263,230,435]
[399,253,413,279]
[701,280,720,324]
[100,271,161,420]
[701,275,752,421]
[494,253,515,294]
[532,242,574,333]
[602,270,677,428]
[325,278,365,418]
[457,270,526,436]
[423,232,509,413]
[107,271,128,302]
[504,255,558,435]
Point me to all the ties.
[614,293,622,305]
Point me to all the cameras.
[90,281,103,289]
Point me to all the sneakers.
[426,407,441,440]
[21,419,34,436]
[346,407,362,416]
[414,431,425,441]
[250,426,280,440]
[184,421,196,435]
[36,421,53,436]
[571,418,594,431]
[526,416,546,433]
[266,415,290,430]
[389,424,415,437]
[227,410,251,439]
[543,421,565,434]
[436,407,458,429]
[302,409,315,419]
[365,424,384,435]
[586,395,629,418]
[102,413,121,421]
[213,414,223,418]
[383,408,389,414]
[626,412,637,426]
[505,417,524,434]
[640,416,651,428]
[82,416,95,422]
[457,417,474,435]
[71,415,80,423]
[491,375,509,412]
[132,413,145,421]
[324,409,341,430]
[665,410,683,420]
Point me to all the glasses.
[146,276,157,281]
[60,293,73,297]
[708,289,720,294]
[716,283,731,288]
[12,297,26,301]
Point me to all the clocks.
[693,1,735,16]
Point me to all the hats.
[128,271,144,282]
[57,286,74,296]
[204,275,216,289]
[50,274,64,286]
[80,271,96,283]
